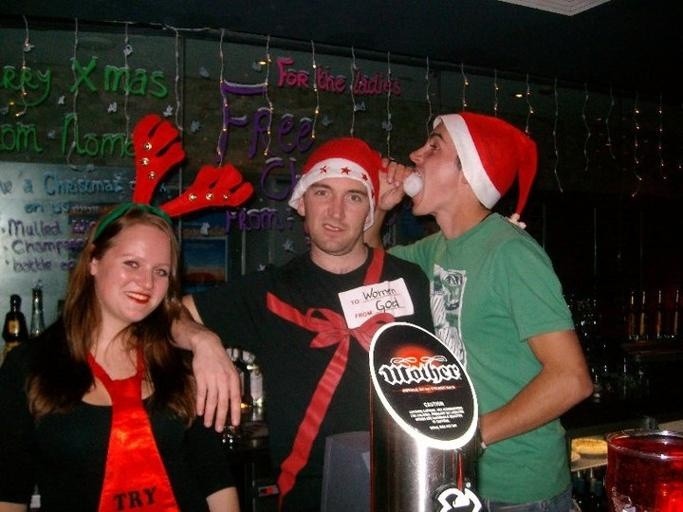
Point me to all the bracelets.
[478,429,488,450]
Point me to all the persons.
[166,136,434,512]
[361,111,595,512]
[1,200,241,512]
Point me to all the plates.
[571,437,607,459]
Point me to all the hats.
[288,137,424,231]
[434,113,539,229]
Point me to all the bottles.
[2,286,46,359]
[563,284,681,406]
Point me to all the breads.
[572,437,608,455]
[570,451,579,462]
